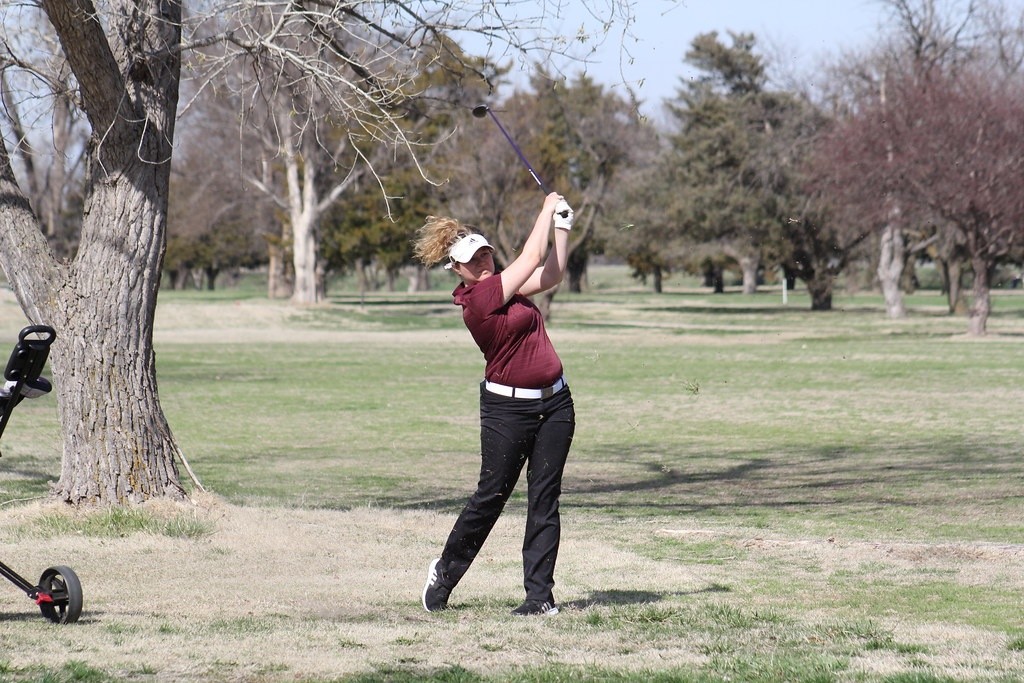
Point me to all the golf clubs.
[472,103,569,219]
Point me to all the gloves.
[553,195,574,231]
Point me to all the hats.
[443,233,495,269]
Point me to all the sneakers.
[509,593,559,620]
[421,558,456,613]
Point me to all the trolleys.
[1,324,84,623]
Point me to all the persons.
[415,191,578,620]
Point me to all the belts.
[485,373,567,399]
